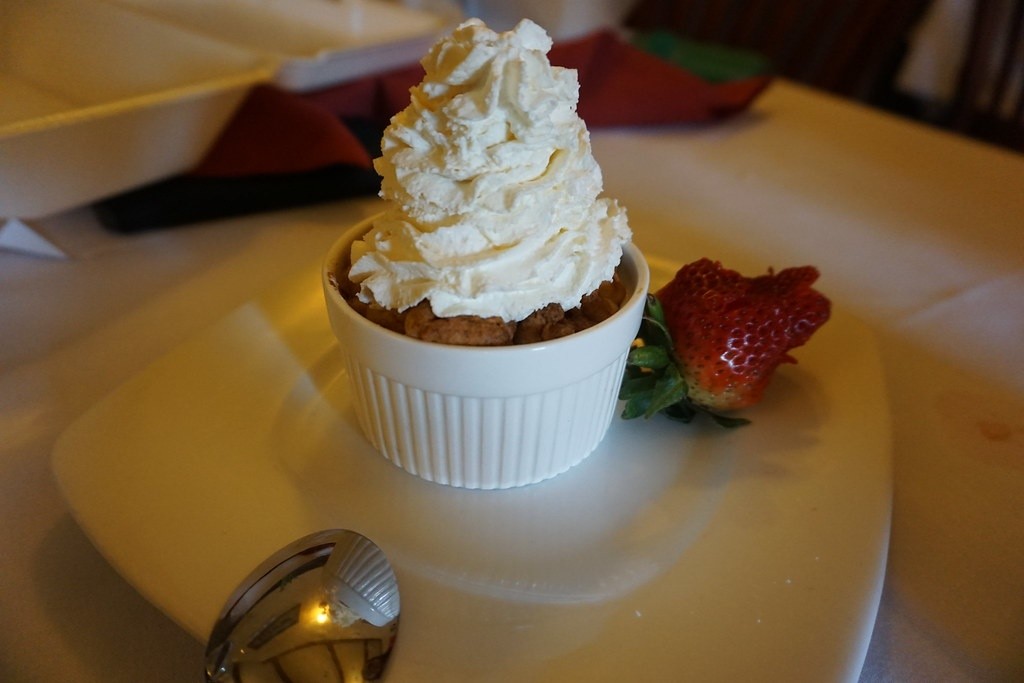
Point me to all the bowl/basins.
[322,209,651,489]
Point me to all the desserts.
[322,18,649,490]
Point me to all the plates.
[50,215,895,683]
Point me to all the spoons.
[205,529,400,683]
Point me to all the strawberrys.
[618,257,831,428]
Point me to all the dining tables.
[1,29,1024,682]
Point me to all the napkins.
[870,266,1023,681]
